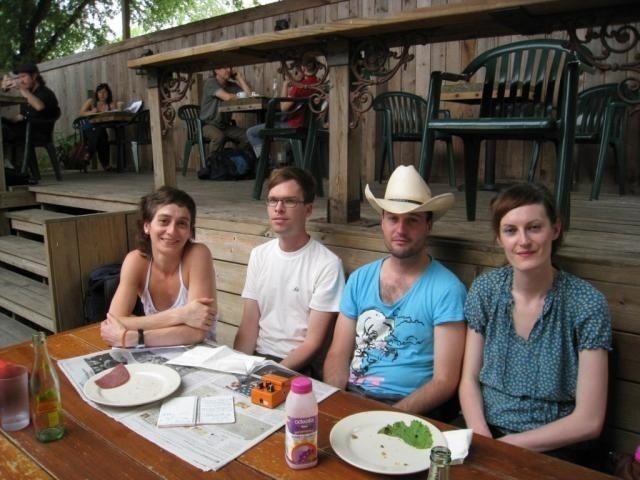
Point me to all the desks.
[1,323,628,480]
[0,96,28,193]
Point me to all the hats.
[365,164,455,222]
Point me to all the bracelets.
[120,327,130,349]
[135,328,147,350]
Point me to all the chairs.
[12,119,62,181]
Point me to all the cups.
[0,364,31,431]
[236,92,247,98]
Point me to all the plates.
[82,362,182,407]
[329,408,444,477]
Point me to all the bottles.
[271,78,280,98]
[30,331,67,444]
[427,445,454,480]
[284,377,319,469]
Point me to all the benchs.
[43,208,640,475]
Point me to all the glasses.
[265,199,304,208]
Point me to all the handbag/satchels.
[211,148,256,181]
[75,146,89,162]
[84,264,143,321]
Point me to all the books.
[154,393,237,427]
[164,341,267,374]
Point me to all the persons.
[246,57,319,174]
[98,183,218,349]
[230,164,344,382]
[0,59,62,186]
[77,82,117,172]
[321,165,470,425]
[458,179,615,471]
[199,66,251,166]
